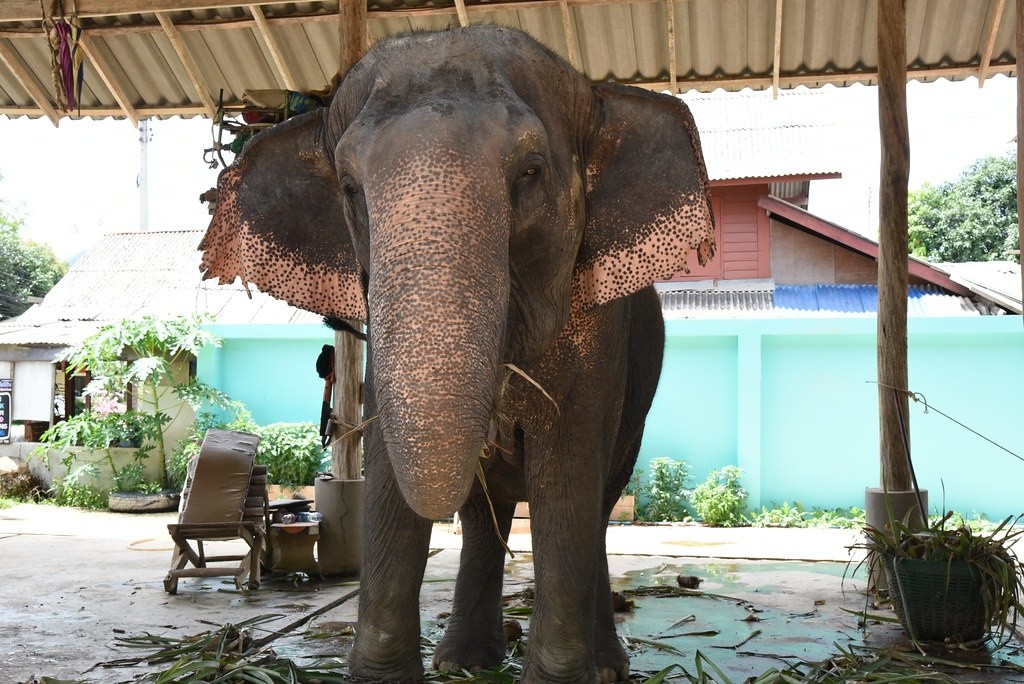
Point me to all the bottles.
[281,513,296,524]
[295,512,322,522]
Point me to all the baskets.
[880,552,995,644]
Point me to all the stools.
[263,522,321,582]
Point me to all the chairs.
[163,464,269,593]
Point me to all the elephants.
[196,23,717,684]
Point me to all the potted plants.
[611,468,643,522]
[840,465,1024,655]
